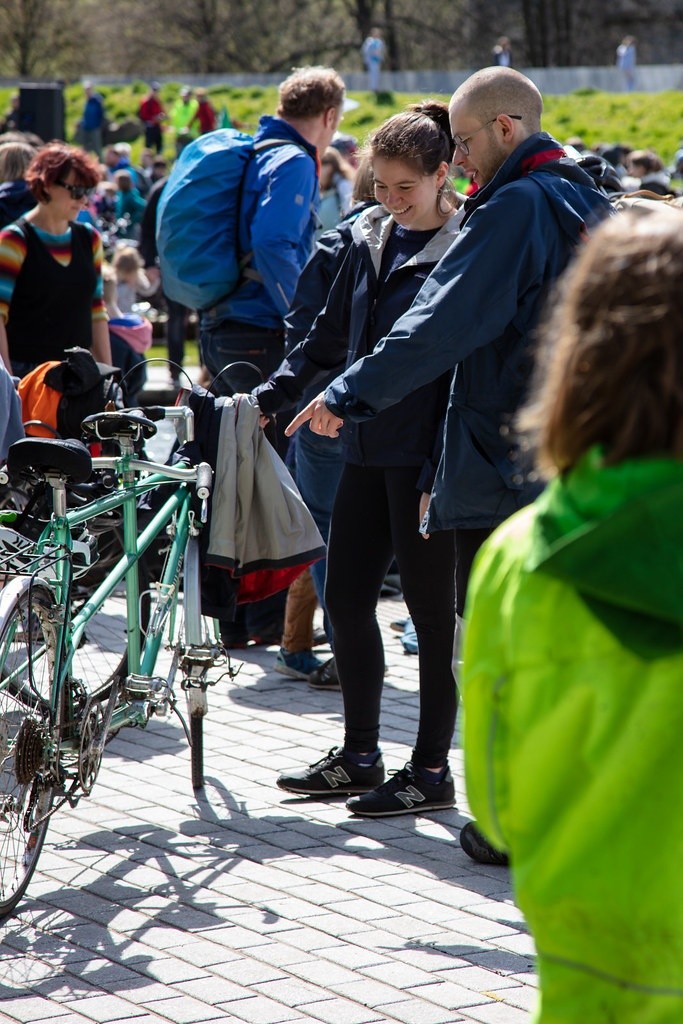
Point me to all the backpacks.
[156,128,317,312]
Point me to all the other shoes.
[273,647,325,680]
[306,656,342,690]
[400,628,418,655]
[460,821,509,867]
[389,617,416,632]
[219,620,254,649]
[249,617,329,647]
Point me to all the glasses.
[453,114,522,156]
[58,179,95,200]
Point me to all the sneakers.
[345,760,457,816]
[276,746,385,794]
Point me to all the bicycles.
[0,358,265,917]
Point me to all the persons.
[180,87,217,135]
[460,200,683,1024]
[247,97,469,818]
[76,79,104,164]
[107,247,161,407]
[139,180,201,388]
[170,84,199,159]
[0,130,115,641]
[4,93,19,130]
[312,129,683,245]
[200,63,345,651]
[140,81,167,154]
[616,36,639,93]
[79,142,165,262]
[284,66,617,869]
[362,22,384,92]
[493,36,513,67]
